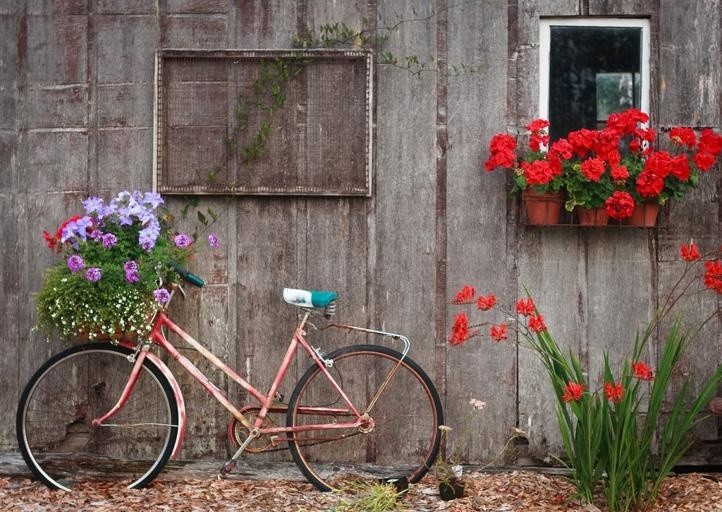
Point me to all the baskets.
[80,301,149,340]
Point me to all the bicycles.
[16,265,443,492]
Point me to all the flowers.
[481,109,722,220]
[32,189,221,344]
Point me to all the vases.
[81,310,138,342]
[521,190,660,227]
